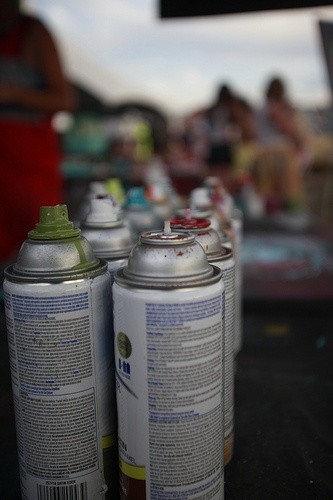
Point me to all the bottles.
[4,204,112,499]
[112,218,224,499]
[80,161,315,473]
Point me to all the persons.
[0,1,75,266]
[94,71,333,235]
